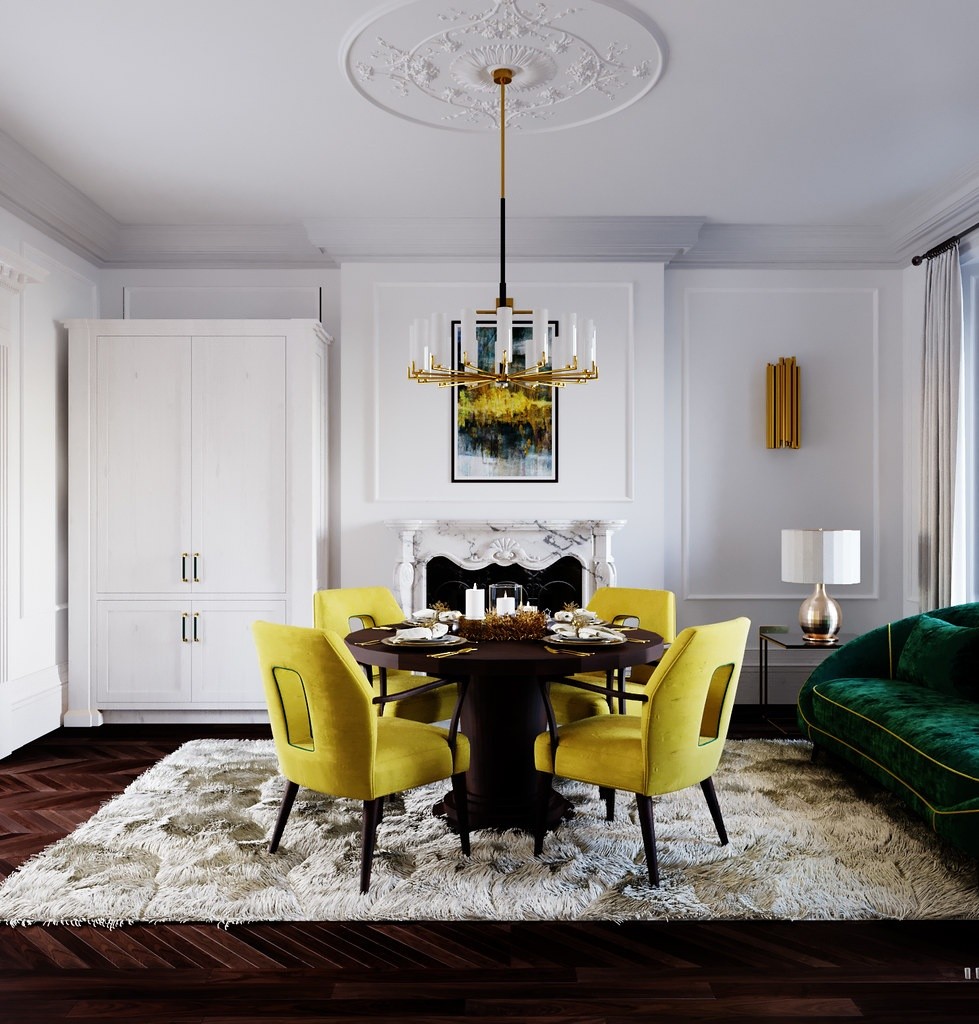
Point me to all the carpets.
[0,740,979,923]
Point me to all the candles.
[522,602,537,611]
[496,590,515,616]
[465,583,486,620]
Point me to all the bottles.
[524,601,537,613]
[496,591,515,617]
[465,583,486,620]
[517,601,524,610]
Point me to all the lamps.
[766,356,801,449]
[781,529,861,641]
[408,68,597,390]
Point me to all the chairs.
[253,587,751,896]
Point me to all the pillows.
[897,614,979,698]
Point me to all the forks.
[544,646,595,657]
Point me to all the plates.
[389,636,461,643]
[544,633,627,645]
[381,635,468,647]
[549,634,618,643]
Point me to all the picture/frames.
[451,320,559,483]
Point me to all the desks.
[760,633,860,734]
[345,620,664,833]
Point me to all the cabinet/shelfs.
[56,317,333,727]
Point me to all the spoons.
[434,648,472,658]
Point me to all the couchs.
[797,603,979,851]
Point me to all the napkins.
[393,620,448,643]
[551,615,616,638]
[555,601,596,621]
[412,600,462,621]
